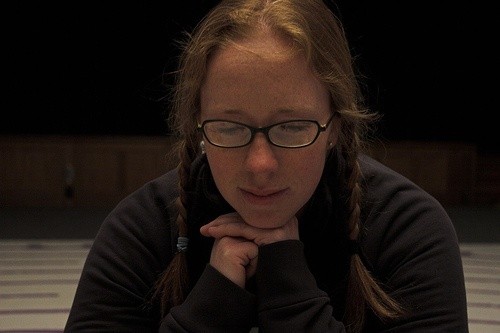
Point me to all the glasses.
[196,109,337,148]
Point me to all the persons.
[64,0,469,333]
